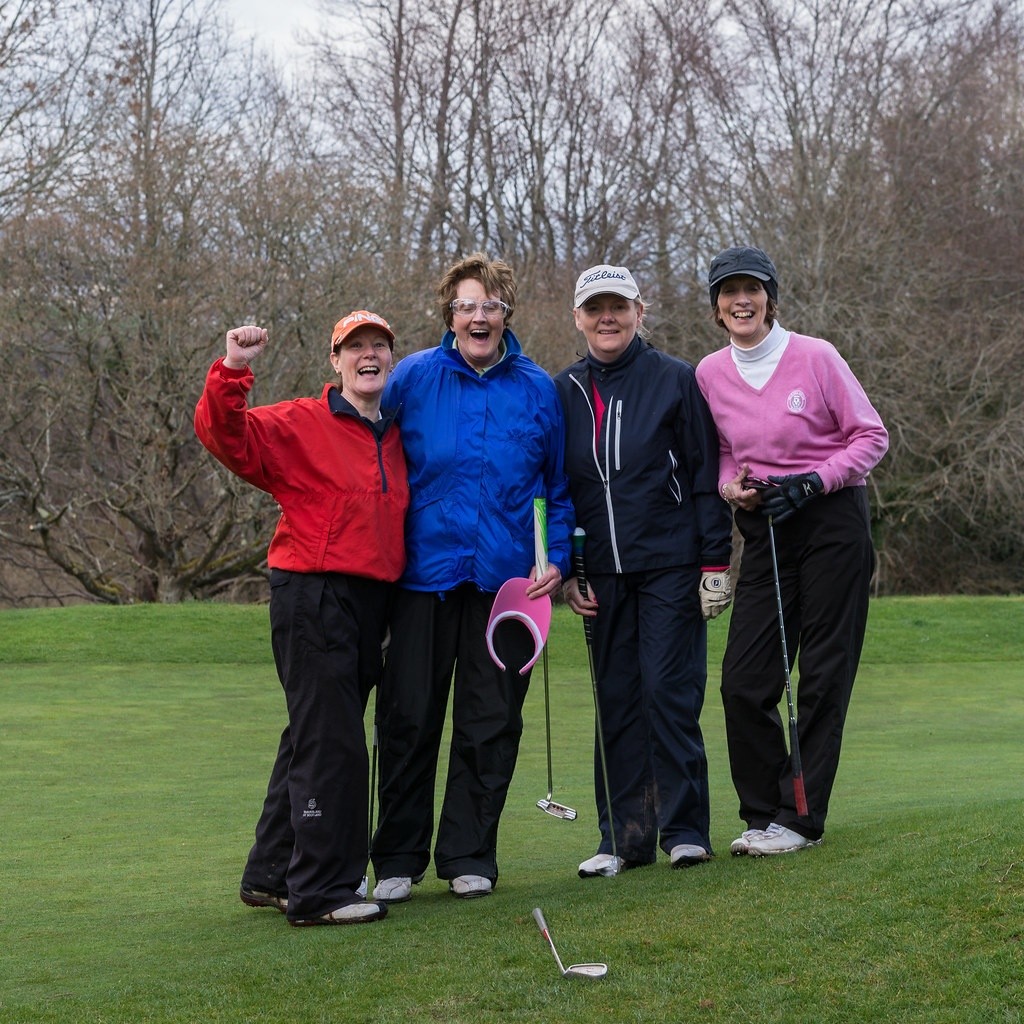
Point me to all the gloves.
[761,471,824,524]
[699,568,733,621]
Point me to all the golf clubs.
[357,723,377,899]
[573,525,621,878]
[530,906,609,980]
[742,474,809,818]
[532,494,580,824]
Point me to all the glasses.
[450,300,509,319]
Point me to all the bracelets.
[722,484,730,504]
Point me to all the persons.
[550,264,732,876]
[693,246,888,854]
[193,310,411,926]
[365,254,573,903]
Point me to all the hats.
[486,577,551,676]
[708,246,779,287]
[710,275,777,310]
[331,310,395,353]
[574,265,642,308]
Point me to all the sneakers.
[287,899,388,927]
[372,875,411,903]
[578,853,628,878]
[670,843,709,870]
[748,823,822,857]
[729,828,766,856]
[240,883,289,913]
[449,875,492,898]
